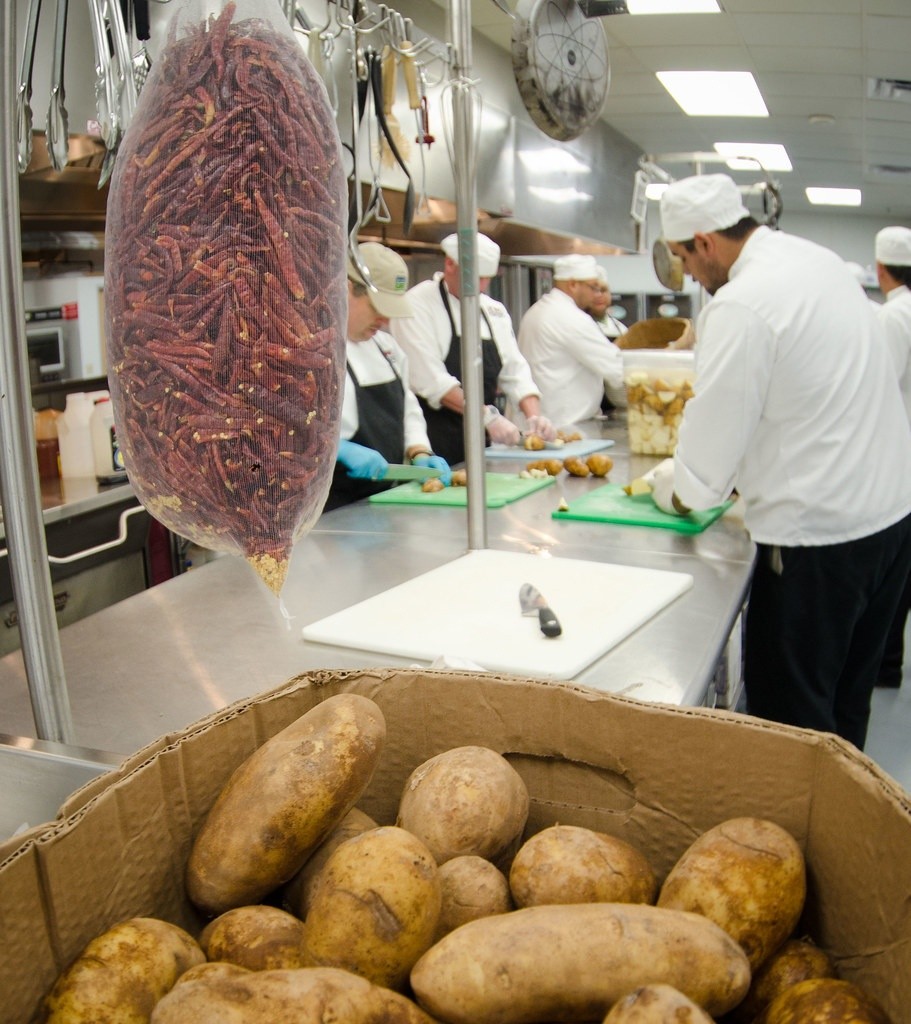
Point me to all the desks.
[0,413,760,851]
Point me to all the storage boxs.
[0,668,911,1024]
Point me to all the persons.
[320,242,450,515]
[651,174,910,752]
[504,253,627,434]
[388,231,555,469]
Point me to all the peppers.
[109,0,348,562]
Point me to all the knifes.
[520,582,562,638]
[371,465,442,481]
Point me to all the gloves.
[337,440,388,482]
[648,471,685,517]
[413,455,452,488]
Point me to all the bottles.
[53,390,128,486]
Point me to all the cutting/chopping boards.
[300,548,694,680]
[485,439,615,461]
[551,481,738,533]
[368,467,556,509]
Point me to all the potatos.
[39,695,896,1024]
[516,430,613,479]
[557,497,569,512]
[421,469,467,493]
[622,478,652,497]
[625,377,696,457]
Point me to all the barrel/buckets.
[619,346,698,457]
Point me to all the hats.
[874,225,911,267]
[552,255,598,281]
[660,173,750,243]
[440,231,500,276]
[345,242,415,319]
[595,265,608,282]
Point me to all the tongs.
[12,1,139,176]
